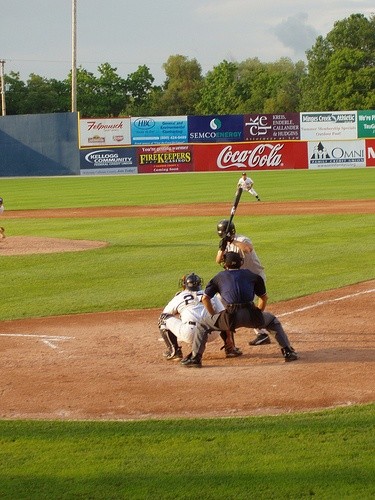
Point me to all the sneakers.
[225,348,242,357]
[167,346,183,361]
[181,354,202,367]
[249,334,270,345]
[280,346,298,362]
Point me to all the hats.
[223,251,241,269]
[242,172,247,176]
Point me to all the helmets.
[185,273,202,291]
[218,220,235,238]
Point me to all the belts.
[225,302,254,308]
[189,322,197,325]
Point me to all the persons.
[235,173,260,201]
[180,251,298,367]
[216,220,270,345]
[158,273,242,359]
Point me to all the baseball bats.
[224,188,243,232]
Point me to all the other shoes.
[257,198,261,201]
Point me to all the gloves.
[225,236,233,242]
[219,238,227,251]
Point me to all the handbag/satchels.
[250,306,265,330]
[213,311,235,333]
